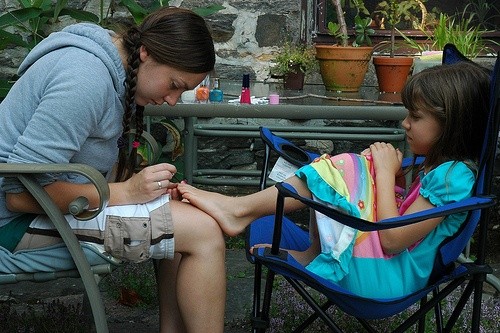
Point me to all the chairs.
[245,43,500,333]
[0,164,129,333]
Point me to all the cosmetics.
[180,74,251,104]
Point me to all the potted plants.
[265,35,320,90]
[315,0,375,92]
[373,0,428,93]
[373,0,500,77]
[98,260,157,313]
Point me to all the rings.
[157,181,163,190]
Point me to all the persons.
[0,6,226,333]
[176,62,495,300]
